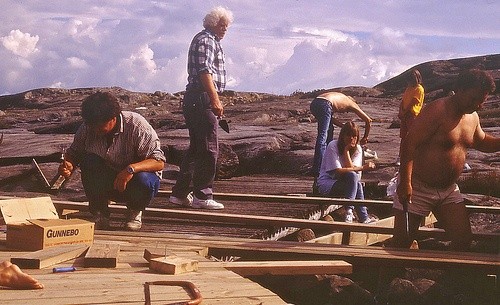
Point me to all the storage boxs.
[0,195,95,251]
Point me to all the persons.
[394,69,424,166]
[58,91,167,230]
[309,91,372,178]
[168,5,234,210]
[316,121,379,224]
[389,68,500,251]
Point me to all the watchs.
[127,165,135,174]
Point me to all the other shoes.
[344,211,356,223]
[123,208,142,232]
[169,191,194,207]
[91,216,110,230]
[361,218,379,224]
[190,194,225,210]
